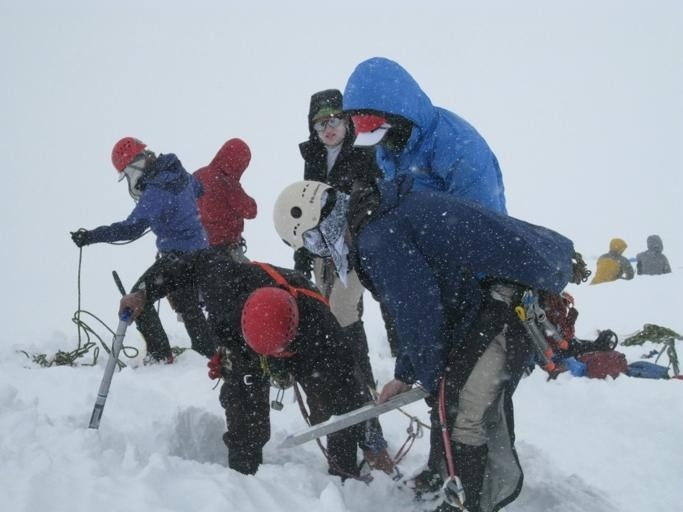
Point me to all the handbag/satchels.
[578,348,628,378]
[628,359,669,381]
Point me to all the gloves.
[68,231,90,248]
[117,287,148,325]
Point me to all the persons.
[292,88,378,399]
[67,136,215,368]
[336,56,506,214]
[118,246,406,487]
[190,139,257,342]
[587,236,635,287]
[636,233,671,276]
[272,175,578,511]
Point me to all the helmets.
[240,286,300,357]
[271,180,337,252]
[110,136,147,173]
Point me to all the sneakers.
[228,445,263,476]
[327,446,360,482]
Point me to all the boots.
[397,427,455,499]
[360,447,394,477]
[429,439,488,511]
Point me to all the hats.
[351,111,393,148]
[311,105,344,121]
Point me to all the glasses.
[312,115,345,132]
